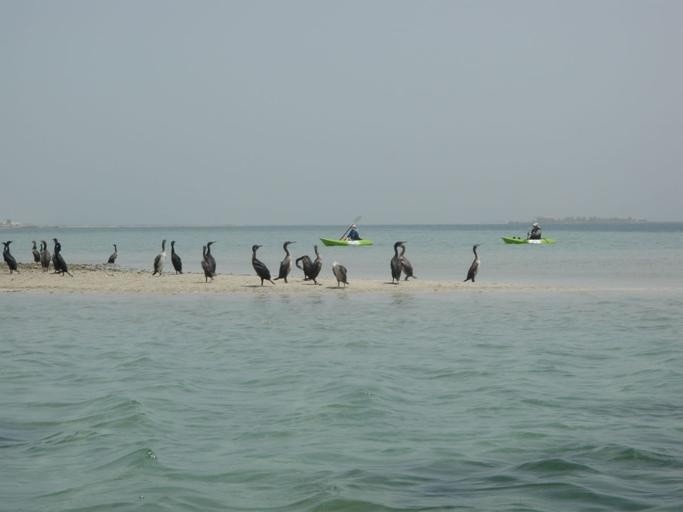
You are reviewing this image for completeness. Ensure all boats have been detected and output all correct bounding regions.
[501,235,557,245]
[319,237,373,247]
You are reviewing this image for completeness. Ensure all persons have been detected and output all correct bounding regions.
[527,222,541,240]
[344,224,361,240]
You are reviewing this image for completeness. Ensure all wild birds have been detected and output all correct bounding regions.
[250,240,322,287]
[6,240,18,271]
[391,241,407,284]
[206,241,217,274]
[1,242,15,274]
[462,243,482,282]
[400,245,418,281]
[201,245,217,282]
[171,240,184,275]
[105,244,118,266]
[31,238,75,277]
[152,239,167,277]
[333,260,350,288]
